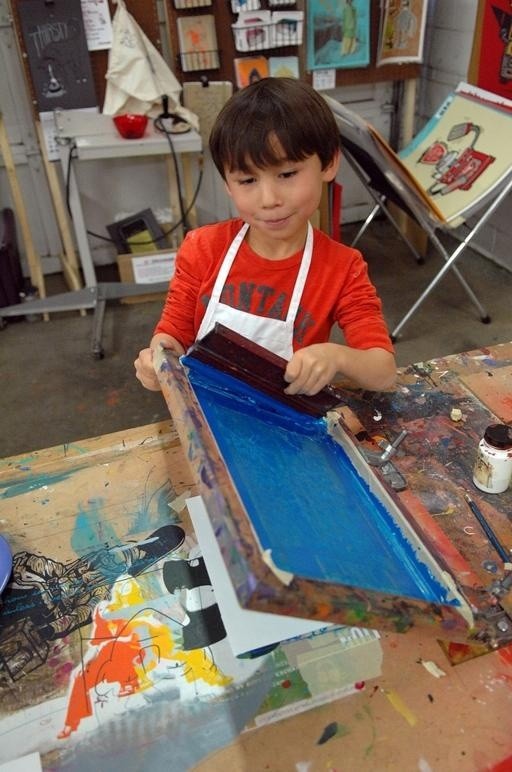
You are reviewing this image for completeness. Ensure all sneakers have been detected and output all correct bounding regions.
[126,525,186,578]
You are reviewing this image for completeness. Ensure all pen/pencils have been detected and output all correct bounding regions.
[463,493,508,563]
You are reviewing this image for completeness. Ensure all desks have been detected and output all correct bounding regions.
[3,336,512,772]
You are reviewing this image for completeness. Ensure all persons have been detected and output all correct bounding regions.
[134,76,397,397]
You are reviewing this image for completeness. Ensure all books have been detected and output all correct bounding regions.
[177,14,221,73]
[173,0,212,9]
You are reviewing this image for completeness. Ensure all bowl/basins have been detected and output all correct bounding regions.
[113,112,150,139]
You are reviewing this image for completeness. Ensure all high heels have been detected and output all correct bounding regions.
[118,679,140,697]
[56,723,77,739]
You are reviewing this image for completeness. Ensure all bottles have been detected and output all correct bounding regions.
[474,422,512,495]
[19,276,41,323]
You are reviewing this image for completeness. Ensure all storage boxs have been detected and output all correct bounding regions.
[117,223,184,304]
